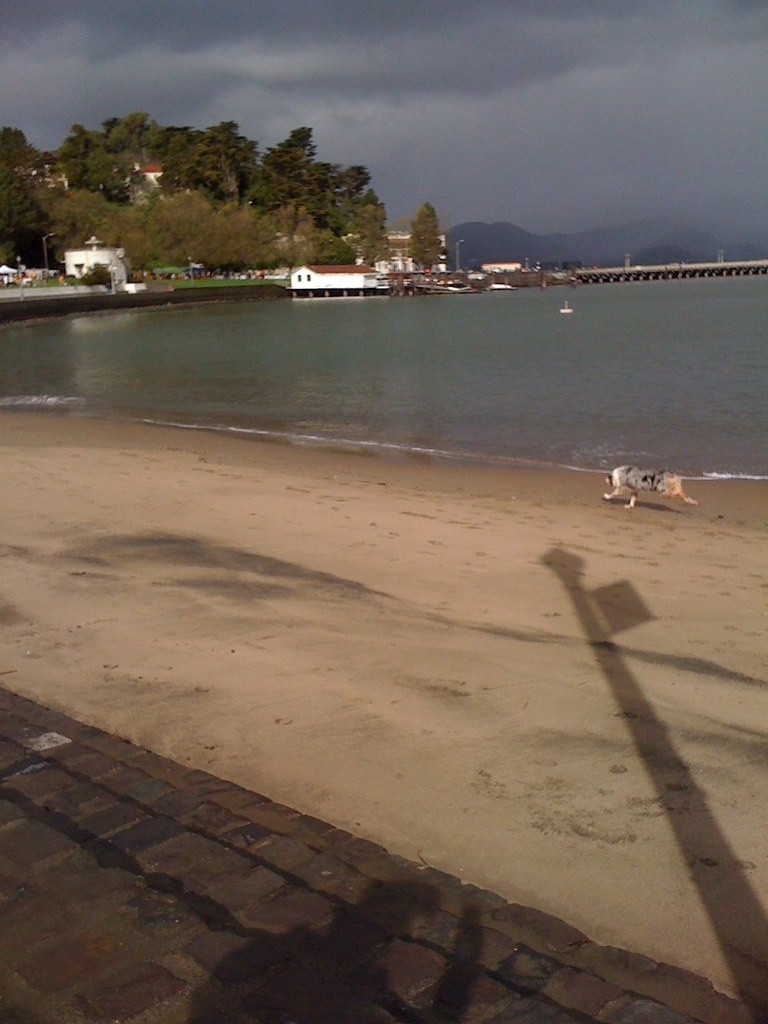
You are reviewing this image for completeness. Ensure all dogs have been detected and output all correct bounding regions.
[601,465,699,510]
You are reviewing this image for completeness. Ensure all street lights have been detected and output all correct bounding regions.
[455,239,465,270]
[41,232,55,286]
